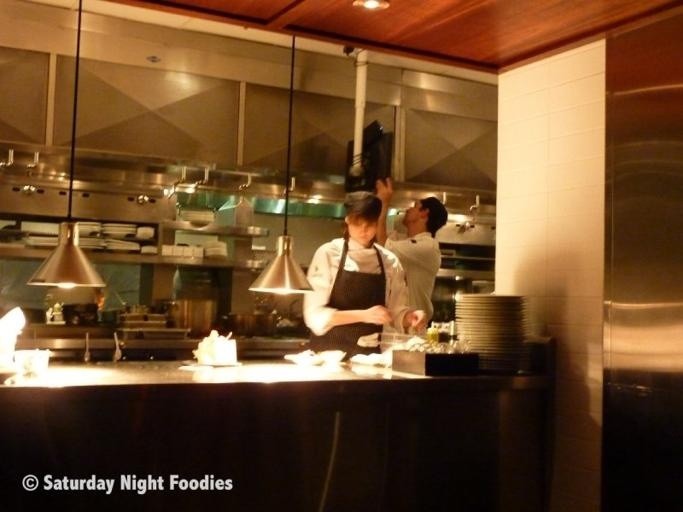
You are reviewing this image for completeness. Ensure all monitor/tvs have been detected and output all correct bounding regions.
[345,132,393,192]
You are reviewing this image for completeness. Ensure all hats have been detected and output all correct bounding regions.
[344,191,381,213]
[424,197,447,230]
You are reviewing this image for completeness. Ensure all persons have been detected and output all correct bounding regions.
[301,191,428,360]
[375,178,447,329]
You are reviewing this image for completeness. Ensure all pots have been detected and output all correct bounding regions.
[164,299,277,339]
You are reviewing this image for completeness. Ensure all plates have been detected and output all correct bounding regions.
[203,241,227,259]
[454,293,527,373]
[20,222,140,252]
[179,212,214,227]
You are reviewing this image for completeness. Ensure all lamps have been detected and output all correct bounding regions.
[25,0,104,292]
[245,31,318,297]
